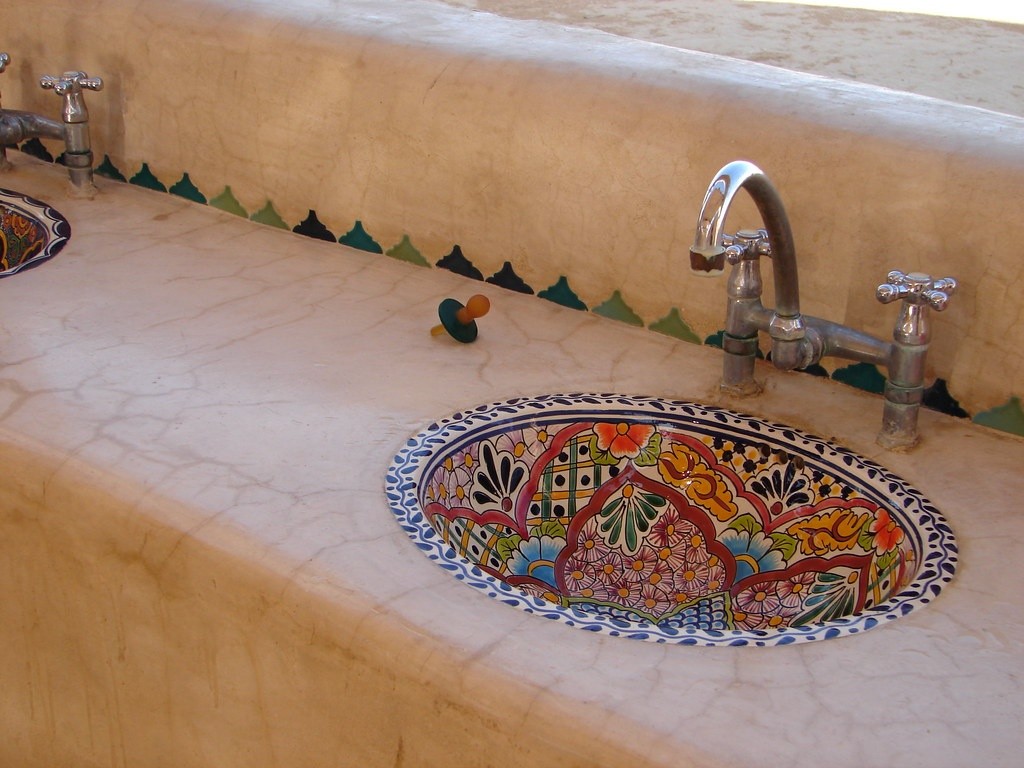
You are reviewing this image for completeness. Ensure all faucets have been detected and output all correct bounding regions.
[688,159,808,373]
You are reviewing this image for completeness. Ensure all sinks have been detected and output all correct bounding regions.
[0,187,72,278]
[384,391,960,647]
